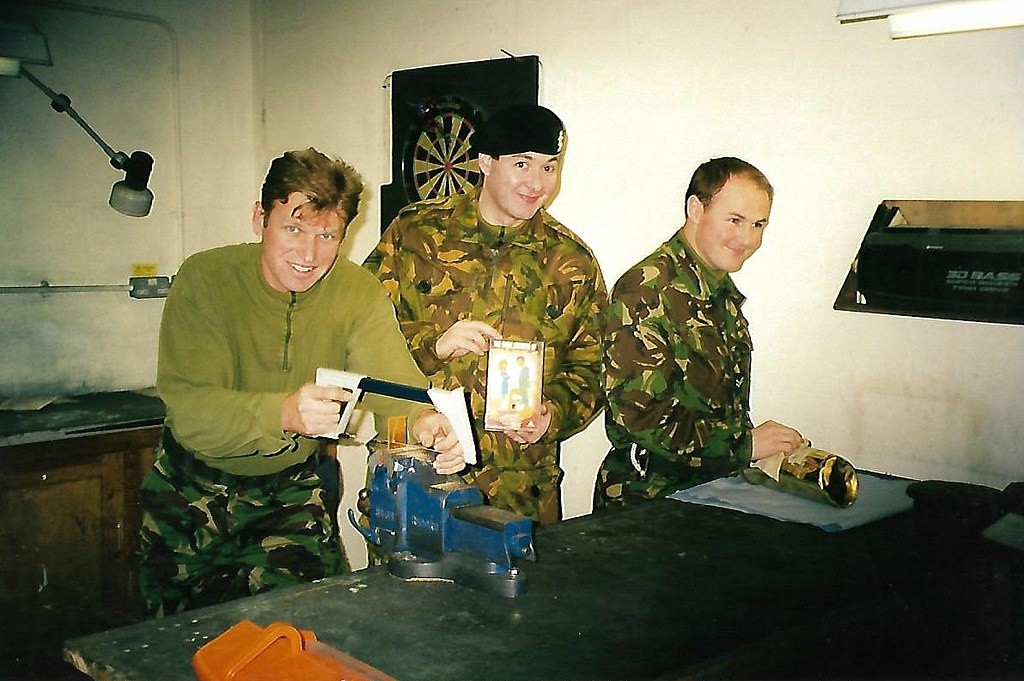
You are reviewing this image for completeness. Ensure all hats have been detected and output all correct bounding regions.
[468,104,565,157]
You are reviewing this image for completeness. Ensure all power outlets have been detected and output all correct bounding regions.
[129,277,169,299]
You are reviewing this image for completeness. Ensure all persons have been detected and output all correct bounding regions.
[128,145,472,626]
[358,103,610,571]
[591,153,804,515]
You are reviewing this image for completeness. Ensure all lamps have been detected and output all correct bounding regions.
[0,58,154,217]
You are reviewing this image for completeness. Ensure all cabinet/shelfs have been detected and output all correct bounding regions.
[1,427,163,652]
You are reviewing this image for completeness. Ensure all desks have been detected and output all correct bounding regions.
[59,466,1024,681]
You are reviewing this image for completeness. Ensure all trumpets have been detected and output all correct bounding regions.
[746,443,859,508]
